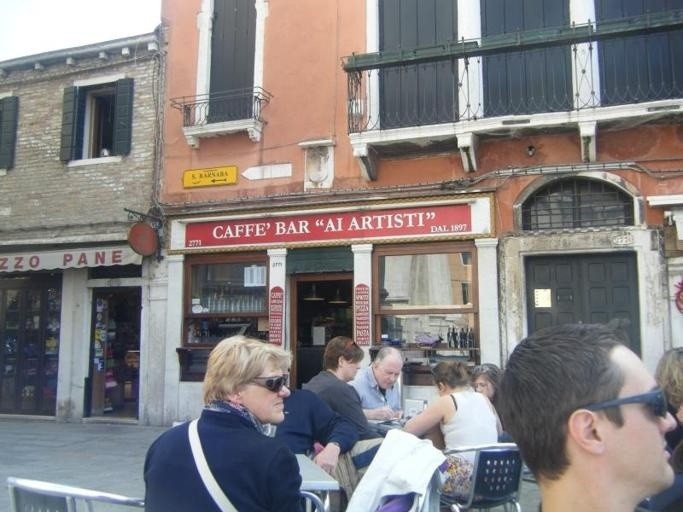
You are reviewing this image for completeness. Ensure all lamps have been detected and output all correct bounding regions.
[328,287,347,303]
[303,283,324,300]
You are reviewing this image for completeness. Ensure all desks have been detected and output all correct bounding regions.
[295,453,339,512]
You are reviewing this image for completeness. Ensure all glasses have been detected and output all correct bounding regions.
[248,373,290,393]
[561,385,669,421]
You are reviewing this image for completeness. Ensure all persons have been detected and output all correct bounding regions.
[297,336,383,441]
[490,319,679,510]
[635,344,683,511]
[468,362,513,443]
[400,358,502,510]
[342,343,406,425]
[274,382,384,511]
[446,325,478,348]
[138,333,305,510]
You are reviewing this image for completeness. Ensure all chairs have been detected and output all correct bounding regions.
[451,443,523,512]
